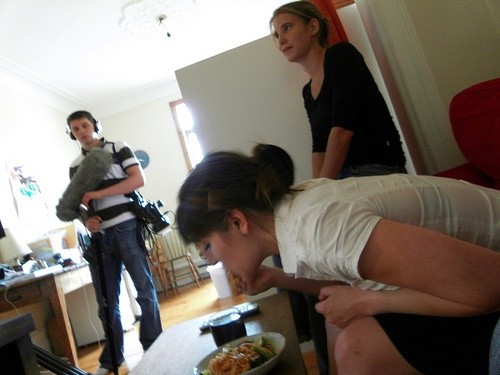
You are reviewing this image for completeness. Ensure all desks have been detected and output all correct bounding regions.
[0,246,149,375]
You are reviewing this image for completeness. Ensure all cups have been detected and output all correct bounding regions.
[207,306,247,348]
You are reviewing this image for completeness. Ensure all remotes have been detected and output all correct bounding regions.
[200,301,260,330]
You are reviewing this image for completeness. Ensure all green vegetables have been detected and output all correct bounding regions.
[251,335,276,361]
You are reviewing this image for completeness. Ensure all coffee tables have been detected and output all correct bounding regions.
[126,291,307,374]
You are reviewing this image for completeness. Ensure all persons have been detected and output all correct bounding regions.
[176,144,500,375]
[66,109,162,375]
[270,1,408,178]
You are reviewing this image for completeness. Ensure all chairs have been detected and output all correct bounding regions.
[152,230,204,298]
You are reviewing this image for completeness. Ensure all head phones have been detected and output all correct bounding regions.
[70,119,99,141]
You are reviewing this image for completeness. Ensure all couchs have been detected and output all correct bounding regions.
[431,77,500,190]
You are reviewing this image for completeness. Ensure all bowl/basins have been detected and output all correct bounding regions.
[193,331,285,375]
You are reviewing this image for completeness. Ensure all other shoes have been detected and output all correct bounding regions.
[103,362,130,375]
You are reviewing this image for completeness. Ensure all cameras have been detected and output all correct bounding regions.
[145,203,171,233]
